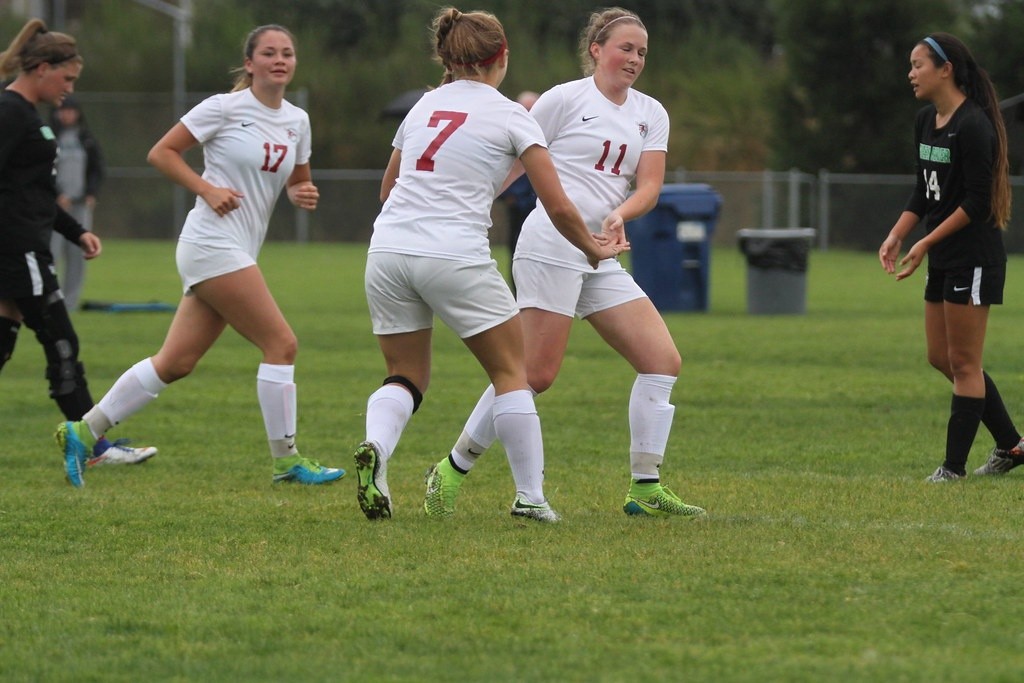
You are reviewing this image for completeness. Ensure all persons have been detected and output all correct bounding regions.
[0,17,158,469]
[54,23,347,486]
[877,32,1024,483]
[420,6,711,522]
[354,7,561,528]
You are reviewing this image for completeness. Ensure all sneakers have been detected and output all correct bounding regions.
[53,419,97,489]
[84,437,158,469]
[353,439,392,520]
[973,438,1024,475]
[623,483,707,519]
[272,457,346,485]
[511,495,563,524]
[925,466,968,483]
[423,463,456,521]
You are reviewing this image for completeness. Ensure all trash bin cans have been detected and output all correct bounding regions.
[627,183,722,310]
[734,226,814,317]
